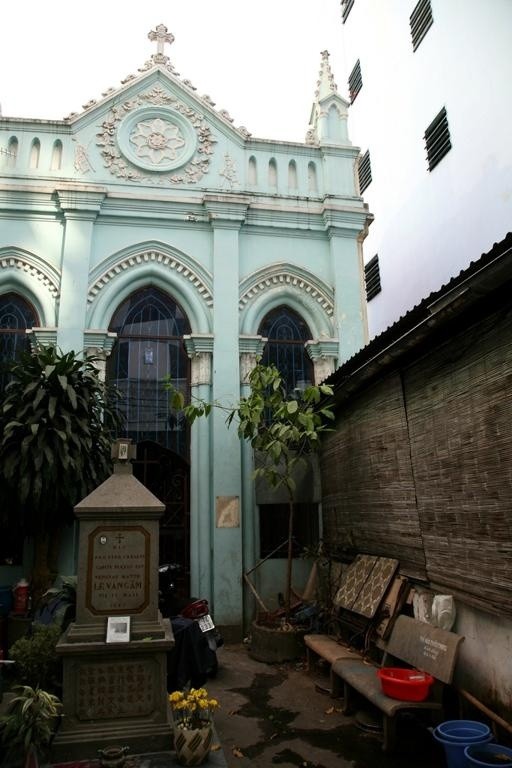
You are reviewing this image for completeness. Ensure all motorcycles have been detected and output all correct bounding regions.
[158,555,225,688]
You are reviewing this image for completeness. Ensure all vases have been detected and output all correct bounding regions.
[172,718,213,768]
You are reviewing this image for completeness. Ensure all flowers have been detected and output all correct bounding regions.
[168,688,223,728]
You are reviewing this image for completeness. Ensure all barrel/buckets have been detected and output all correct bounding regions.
[433,720,512,768]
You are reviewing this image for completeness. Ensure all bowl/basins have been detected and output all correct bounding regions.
[378,666,433,701]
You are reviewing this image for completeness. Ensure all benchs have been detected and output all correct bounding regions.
[331,614,465,750]
[304,617,387,695]
